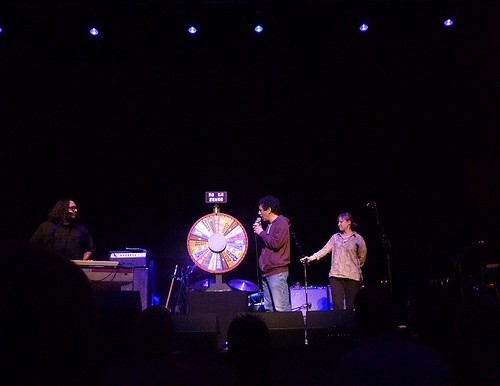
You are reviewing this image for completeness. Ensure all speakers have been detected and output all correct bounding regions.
[173,312,218,349]
[306,309,357,346]
[247,311,305,350]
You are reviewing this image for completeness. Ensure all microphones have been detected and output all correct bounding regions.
[367,201,375,207]
[255,217,261,224]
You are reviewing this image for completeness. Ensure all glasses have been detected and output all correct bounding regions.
[67,206,79,211]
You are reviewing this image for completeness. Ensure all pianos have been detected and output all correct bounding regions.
[73,259,136,291]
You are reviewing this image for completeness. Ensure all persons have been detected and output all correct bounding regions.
[299,213,367,310]
[0,244,500,385]
[252,196,292,313]
[29,198,90,260]
